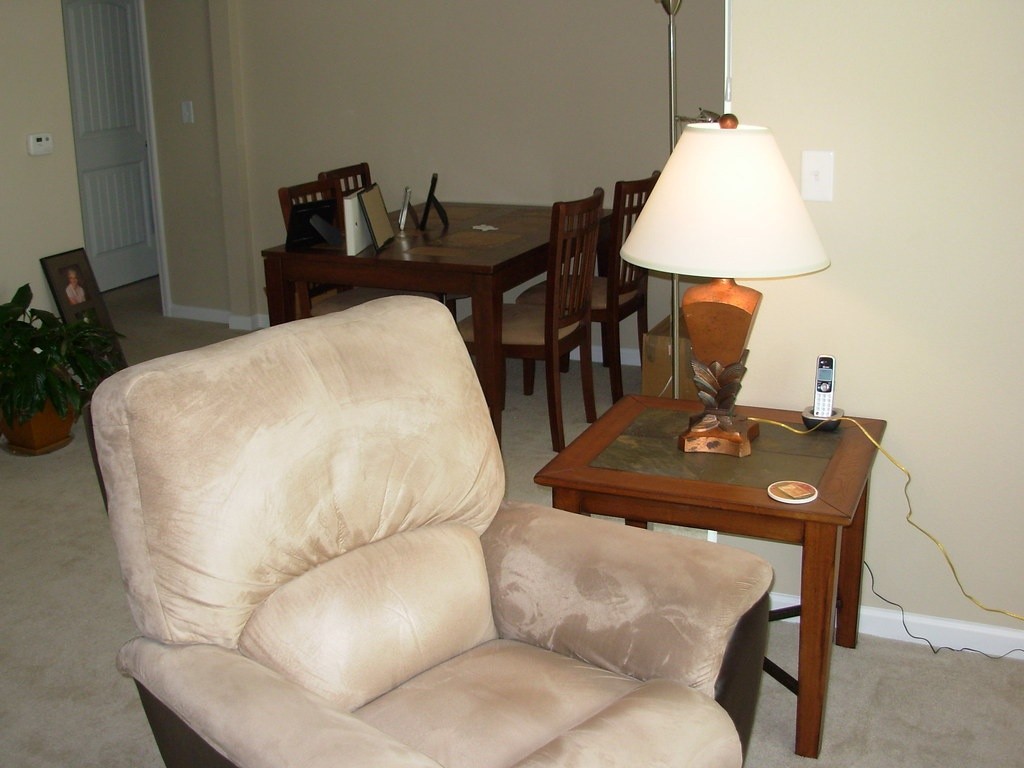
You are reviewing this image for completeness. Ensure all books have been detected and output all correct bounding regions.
[343,186,372,255]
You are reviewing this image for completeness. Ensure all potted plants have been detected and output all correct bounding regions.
[0,285,129,458]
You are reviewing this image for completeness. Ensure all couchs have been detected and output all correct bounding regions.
[82,295,776,768]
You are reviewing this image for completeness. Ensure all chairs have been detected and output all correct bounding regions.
[513,168,661,405]
[277,161,375,244]
[455,187,606,453]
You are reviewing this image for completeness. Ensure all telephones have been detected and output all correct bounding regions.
[801,354,844,432]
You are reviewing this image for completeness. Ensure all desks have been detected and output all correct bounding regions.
[261,197,614,454]
[531,393,887,761]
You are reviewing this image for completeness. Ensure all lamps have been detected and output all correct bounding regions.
[617,113,831,459]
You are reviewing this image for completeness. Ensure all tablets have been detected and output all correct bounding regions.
[358,182,395,252]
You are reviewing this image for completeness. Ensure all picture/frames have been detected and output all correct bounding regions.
[38,248,116,333]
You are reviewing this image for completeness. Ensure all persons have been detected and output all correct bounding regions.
[65,269,85,304]
